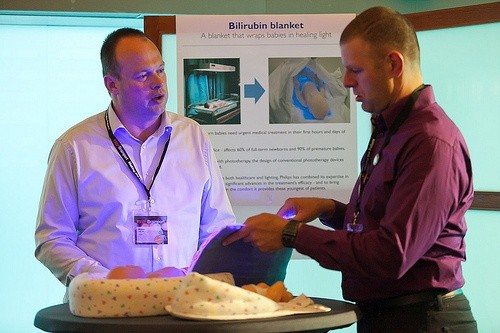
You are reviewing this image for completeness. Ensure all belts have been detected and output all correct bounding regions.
[356,287,463,311]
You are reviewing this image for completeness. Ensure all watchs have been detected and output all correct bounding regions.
[278,219,301,249]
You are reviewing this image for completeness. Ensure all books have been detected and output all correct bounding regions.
[176,223,295,287]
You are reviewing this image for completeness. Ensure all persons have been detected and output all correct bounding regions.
[33,28,236,300]
[291,68,331,120]
[222,5,479,332]
[107,263,189,282]
[239,281,294,302]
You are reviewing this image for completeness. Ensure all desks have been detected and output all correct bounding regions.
[32,295,358,333]
